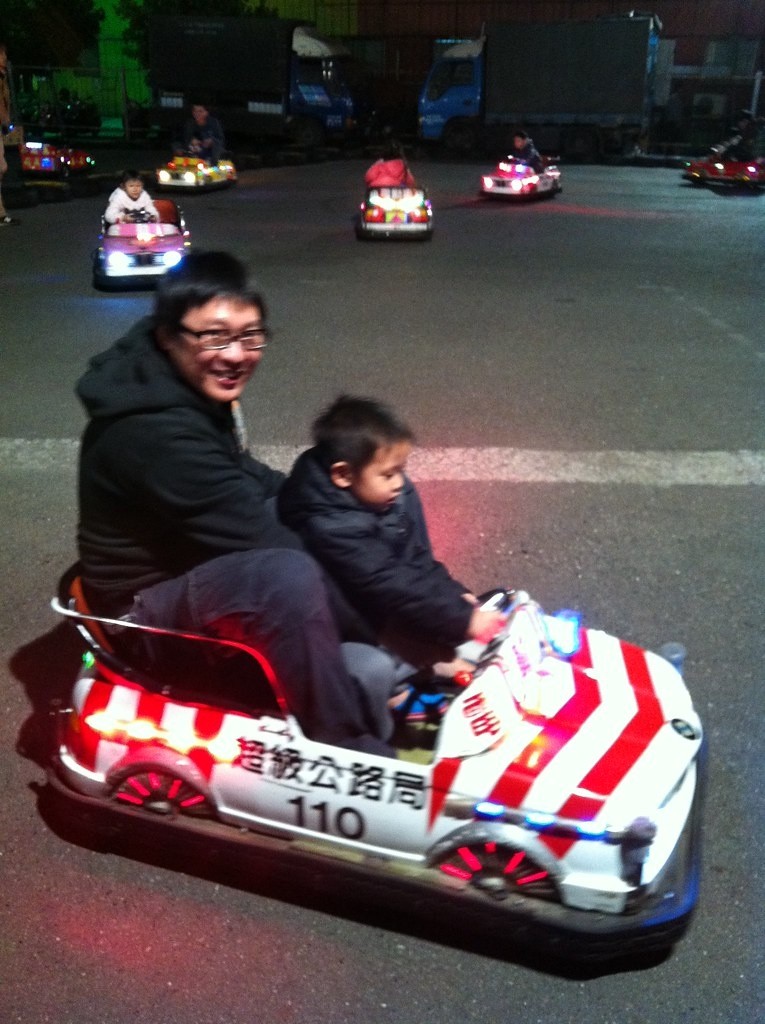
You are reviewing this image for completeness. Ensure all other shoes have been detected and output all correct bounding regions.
[386,685,447,719]
[338,733,397,758]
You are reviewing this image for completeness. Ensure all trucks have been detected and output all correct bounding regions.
[138,13,379,144]
[417,9,662,157]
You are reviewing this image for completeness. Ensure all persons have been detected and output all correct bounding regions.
[505,131,543,173]
[74,252,400,759]
[175,101,222,165]
[276,389,507,730]
[716,105,765,162]
[103,166,160,224]
[365,143,417,190]
[0,46,22,228]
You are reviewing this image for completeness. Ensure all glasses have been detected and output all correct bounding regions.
[179,324,272,350]
